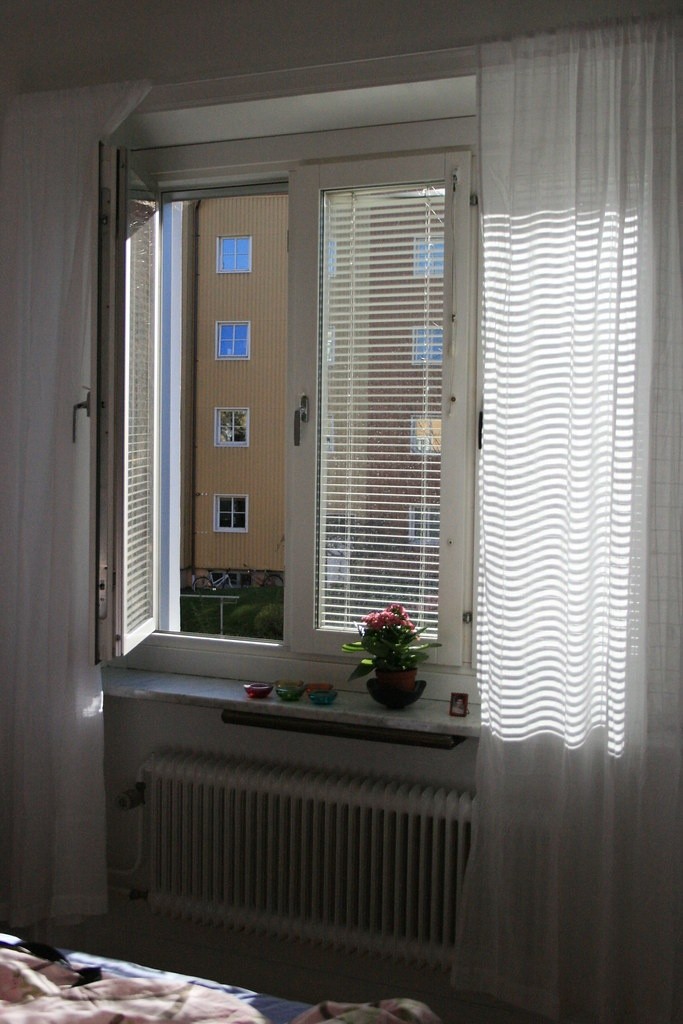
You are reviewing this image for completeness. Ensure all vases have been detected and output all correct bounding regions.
[366,669,426,710]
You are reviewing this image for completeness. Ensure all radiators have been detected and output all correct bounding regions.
[116,746,555,993]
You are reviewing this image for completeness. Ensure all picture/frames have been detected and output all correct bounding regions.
[449,690,468,719]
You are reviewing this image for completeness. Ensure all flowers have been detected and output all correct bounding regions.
[342,602,442,682]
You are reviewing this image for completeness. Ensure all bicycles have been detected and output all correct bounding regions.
[241,563,284,587]
[191,566,242,593]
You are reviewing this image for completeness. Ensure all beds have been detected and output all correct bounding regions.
[0,934,446,1024]
